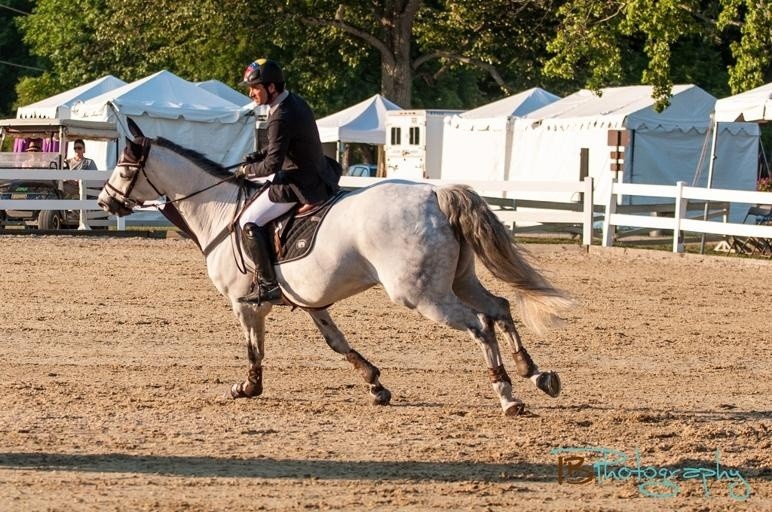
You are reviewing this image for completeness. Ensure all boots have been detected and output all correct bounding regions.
[237,222,284,305]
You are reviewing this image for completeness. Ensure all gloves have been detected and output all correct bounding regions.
[234,150,262,179]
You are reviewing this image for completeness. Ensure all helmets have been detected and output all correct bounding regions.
[238,58,283,86]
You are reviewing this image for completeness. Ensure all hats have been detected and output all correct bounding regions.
[24,141,44,151]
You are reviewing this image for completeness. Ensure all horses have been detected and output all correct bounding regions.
[97,116,572,416]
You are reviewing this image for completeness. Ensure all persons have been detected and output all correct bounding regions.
[232,58,343,305]
[24,140,47,220]
[61,138,112,229]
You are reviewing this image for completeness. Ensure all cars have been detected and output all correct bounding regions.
[347,162,379,177]
